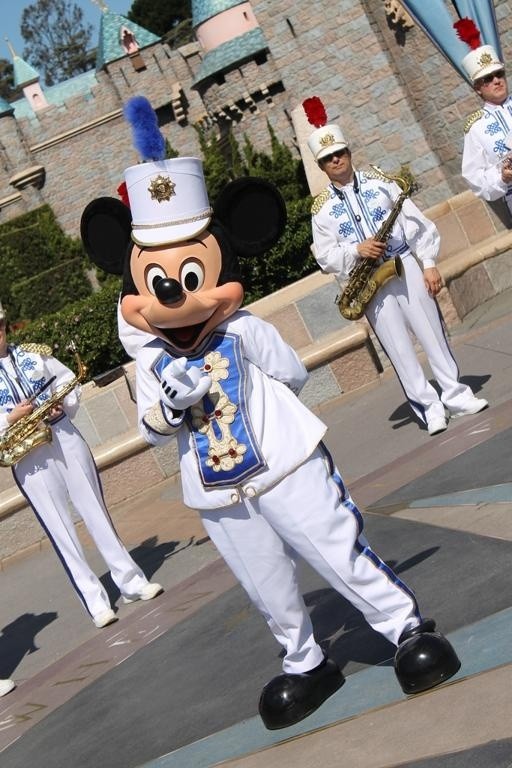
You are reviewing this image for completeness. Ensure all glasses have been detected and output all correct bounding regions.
[476,68,505,89]
[321,148,348,164]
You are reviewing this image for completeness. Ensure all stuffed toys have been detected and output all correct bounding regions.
[77,97,463,733]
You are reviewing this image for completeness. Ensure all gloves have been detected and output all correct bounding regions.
[157,354,214,413]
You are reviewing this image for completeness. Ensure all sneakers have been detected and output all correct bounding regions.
[425,403,450,437]
[447,396,489,418]
[0,677,16,698]
[122,581,165,605]
[92,608,119,629]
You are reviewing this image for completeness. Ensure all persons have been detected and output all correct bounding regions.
[315,145,490,438]
[462,66,511,224]
[0,302,164,631]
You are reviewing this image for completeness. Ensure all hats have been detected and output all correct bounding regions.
[122,154,218,249]
[303,123,352,164]
[459,43,506,86]
[0,303,6,321]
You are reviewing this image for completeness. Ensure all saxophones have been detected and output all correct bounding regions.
[335,162,420,320]
[0,338,85,467]
